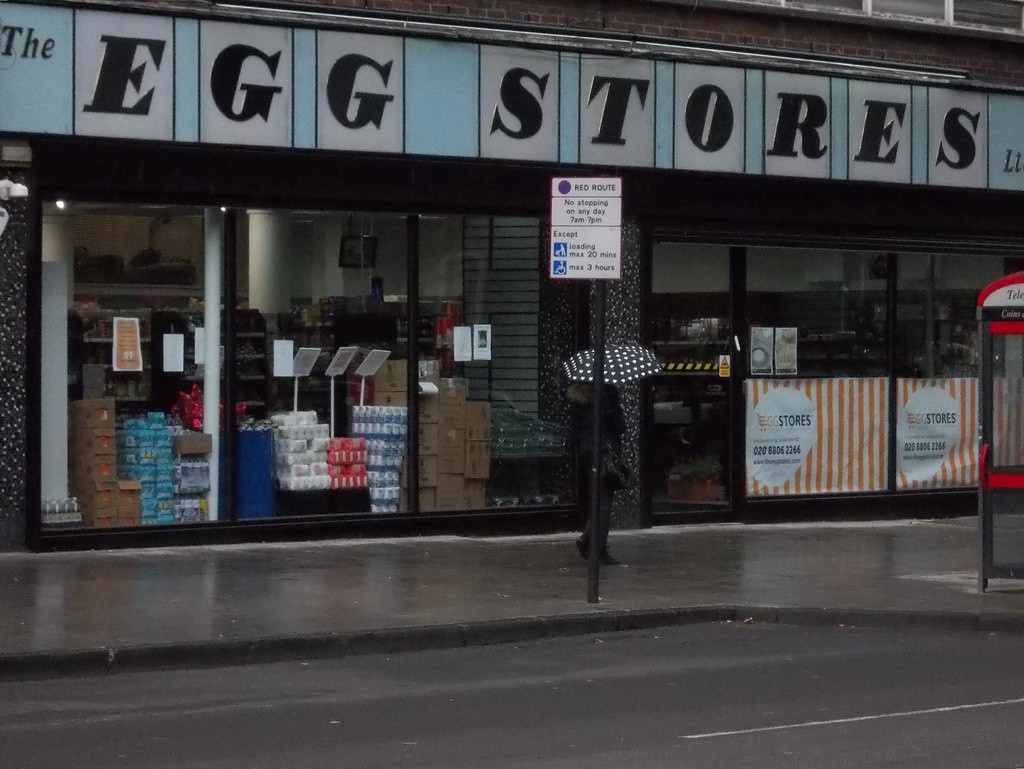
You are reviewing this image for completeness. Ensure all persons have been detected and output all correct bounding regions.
[569,380,629,566]
[67,308,87,402]
[832,320,923,378]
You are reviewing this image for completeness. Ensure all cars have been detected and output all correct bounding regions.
[654,390,724,427]
[907,342,976,376]
[309,379,370,420]
[832,342,886,375]
[469,383,570,460]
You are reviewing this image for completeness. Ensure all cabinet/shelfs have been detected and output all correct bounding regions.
[652,338,884,442]
[72,283,444,407]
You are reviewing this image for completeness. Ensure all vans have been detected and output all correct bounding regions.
[778,343,828,361]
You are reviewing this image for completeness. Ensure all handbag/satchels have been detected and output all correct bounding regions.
[606,450,628,492]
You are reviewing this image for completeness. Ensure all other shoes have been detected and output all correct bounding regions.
[576,536,590,559]
[599,546,620,565]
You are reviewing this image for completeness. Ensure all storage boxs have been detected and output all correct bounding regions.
[374,359,440,391]
[399,456,437,488]
[439,382,467,406]
[467,401,491,441]
[438,407,466,429]
[397,487,435,512]
[117,481,142,507]
[117,507,140,527]
[69,428,116,455]
[80,509,117,528]
[439,431,465,475]
[172,431,212,455]
[404,424,439,457]
[68,399,116,430]
[435,475,464,511]
[68,483,117,508]
[465,441,490,479]
[373,390,439,423]
[464,478,486,510]
[68,456,116,483]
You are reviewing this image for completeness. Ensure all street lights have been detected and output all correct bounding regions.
[840,284,850,329]
[871,255,893,373]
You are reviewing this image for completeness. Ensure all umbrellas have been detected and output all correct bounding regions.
[562,342,663,386]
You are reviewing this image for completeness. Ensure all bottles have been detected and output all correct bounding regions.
[42,497,79,526]
